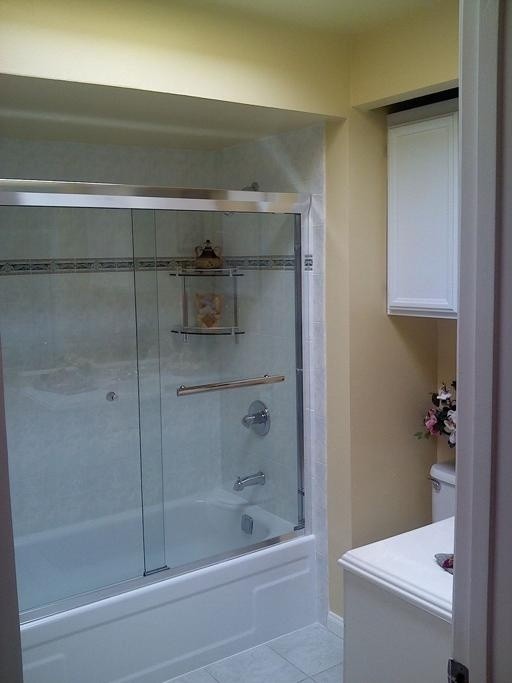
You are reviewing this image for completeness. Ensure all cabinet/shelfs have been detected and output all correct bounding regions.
[380,95,461,321]
[167,263,248,342]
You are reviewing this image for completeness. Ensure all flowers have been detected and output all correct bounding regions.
[410,370,457,450]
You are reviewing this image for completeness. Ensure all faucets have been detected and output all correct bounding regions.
[232,472,267,493]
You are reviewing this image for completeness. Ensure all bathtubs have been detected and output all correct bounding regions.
[9,489,318,682]
